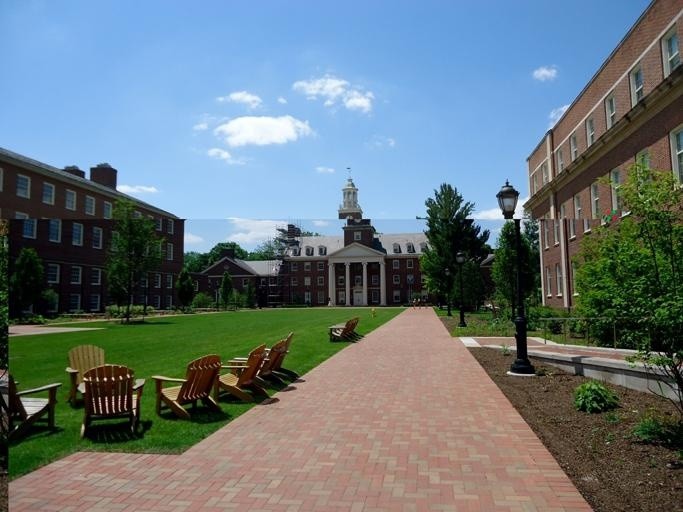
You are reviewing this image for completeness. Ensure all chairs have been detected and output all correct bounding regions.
[327,316,360,342]
[8,331,294,438]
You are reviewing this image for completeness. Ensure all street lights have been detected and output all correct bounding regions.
[442,266,453,317]
[494,175,541,379]
[453,248,473,330]
[437,281,442,312]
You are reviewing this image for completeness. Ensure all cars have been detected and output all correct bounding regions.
[484,303,500,310]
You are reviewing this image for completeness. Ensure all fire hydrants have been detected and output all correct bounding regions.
[369,308,377,319]
